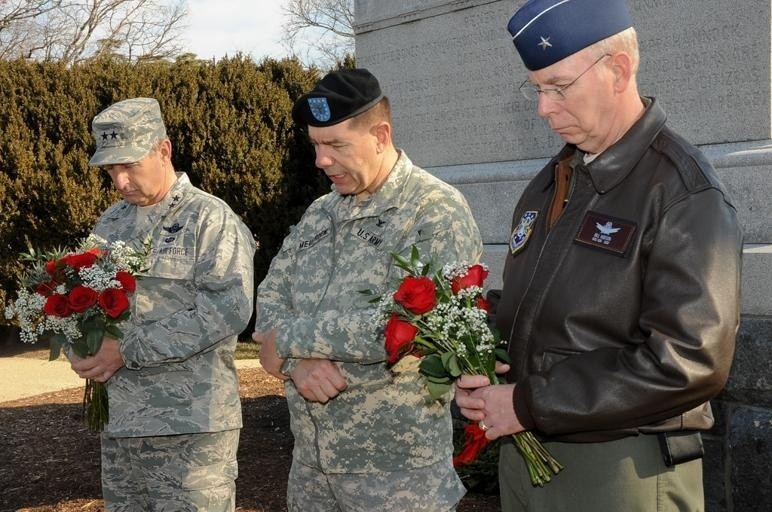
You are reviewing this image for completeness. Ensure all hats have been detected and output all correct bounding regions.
[88,97,167,166]
[507,0,634,72]
[292,68,383,127]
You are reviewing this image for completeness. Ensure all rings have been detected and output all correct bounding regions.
[478,421,488,431]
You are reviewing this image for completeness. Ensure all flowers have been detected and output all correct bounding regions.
[358,244,563,487]
[3,237,157,436]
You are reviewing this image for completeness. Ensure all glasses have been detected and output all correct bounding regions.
[519,54,612,101]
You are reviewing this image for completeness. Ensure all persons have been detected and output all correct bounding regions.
[248,67,488,510]
[452,0,744,512]
[62,94,257,512]
